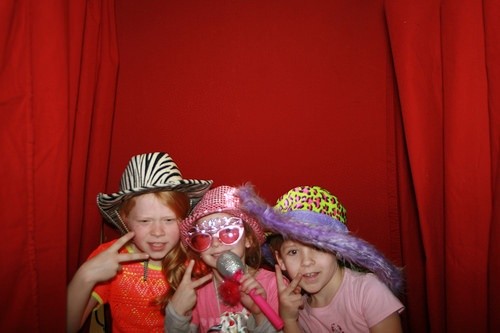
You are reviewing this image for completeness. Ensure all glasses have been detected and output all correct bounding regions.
[185,216,244,251]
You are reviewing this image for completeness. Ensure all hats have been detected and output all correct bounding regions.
[234,182,405,297]
[179,185,265,257]
[97,152,213,235]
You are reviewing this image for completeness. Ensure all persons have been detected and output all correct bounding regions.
[236,185,405,333]
[64,151,214,333]
[150,185,302,333]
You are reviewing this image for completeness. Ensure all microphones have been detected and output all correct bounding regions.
[215,251,285,331]
